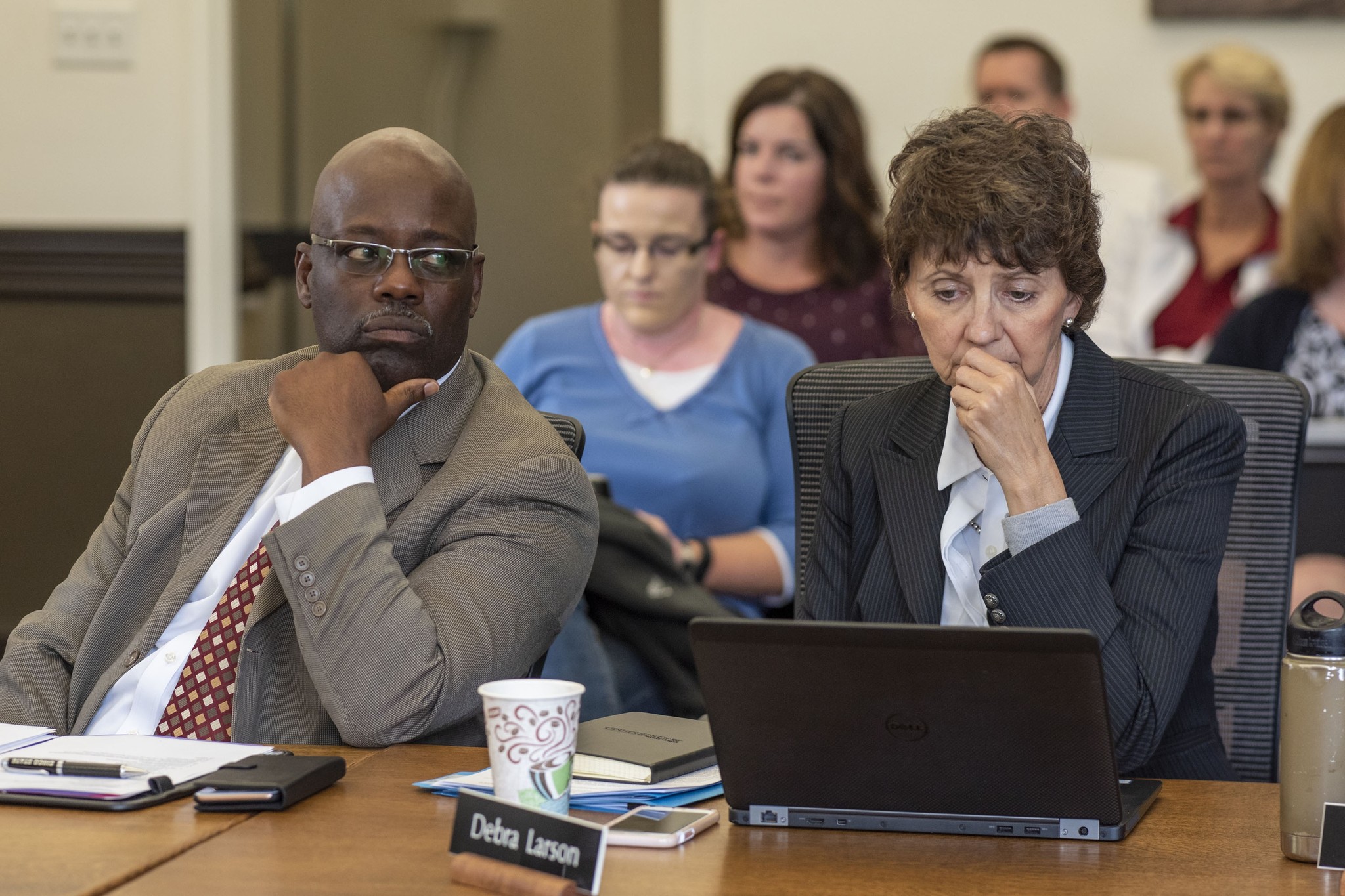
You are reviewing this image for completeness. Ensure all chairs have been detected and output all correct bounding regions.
[524,406,587,681]
[783,354,1315,786]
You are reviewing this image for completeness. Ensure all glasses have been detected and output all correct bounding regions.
[310,233,479,282]
[592,232,710,257]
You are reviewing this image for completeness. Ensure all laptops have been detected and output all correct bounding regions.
[688,618,1162,840]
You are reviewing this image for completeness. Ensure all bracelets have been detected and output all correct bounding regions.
[691,535,710,583]
[681,541,694,581]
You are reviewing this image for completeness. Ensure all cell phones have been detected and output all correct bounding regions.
[603,804,720,848]
[193,754,346,812]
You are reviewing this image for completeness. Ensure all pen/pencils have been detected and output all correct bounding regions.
[0,758,147,778]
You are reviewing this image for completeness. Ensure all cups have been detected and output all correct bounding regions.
[477,677,586,817]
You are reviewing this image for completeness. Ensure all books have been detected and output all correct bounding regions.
[570,711,717,785]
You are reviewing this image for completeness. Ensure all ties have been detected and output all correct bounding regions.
[154,519,280,743]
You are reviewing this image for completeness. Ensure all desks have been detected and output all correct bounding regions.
[0,743,1342,896]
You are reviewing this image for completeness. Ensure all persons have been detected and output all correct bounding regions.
[490,31,1344,723]
[0,125,597,751]
[790,106,1242,782]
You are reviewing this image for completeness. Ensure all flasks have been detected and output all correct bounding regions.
[1275,594,1345,866]
